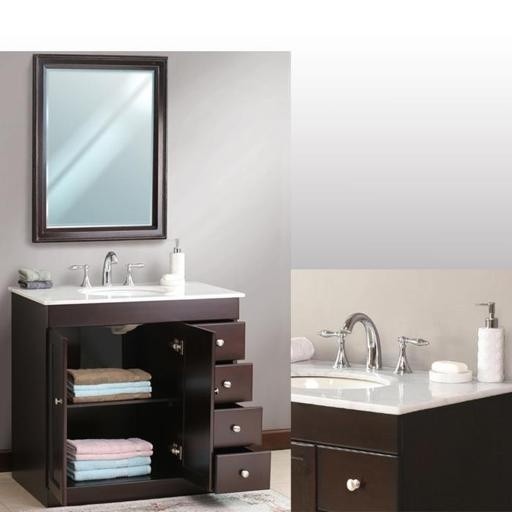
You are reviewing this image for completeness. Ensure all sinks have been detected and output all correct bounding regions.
[290,366,398,390]
[81,287,168,296]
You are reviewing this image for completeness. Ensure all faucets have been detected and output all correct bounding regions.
[339,311,384,369]
[101,251,119,286]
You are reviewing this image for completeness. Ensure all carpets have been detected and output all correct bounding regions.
[37,488,290,512]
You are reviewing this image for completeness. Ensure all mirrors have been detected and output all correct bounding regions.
[31,55,170,242]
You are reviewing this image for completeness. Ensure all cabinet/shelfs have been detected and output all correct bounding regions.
[7,280,273,508]
[291,392,511,512]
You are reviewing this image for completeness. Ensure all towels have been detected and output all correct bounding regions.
[66,366,153,403]
[18,268,52,289]
[65,436,154,482]
